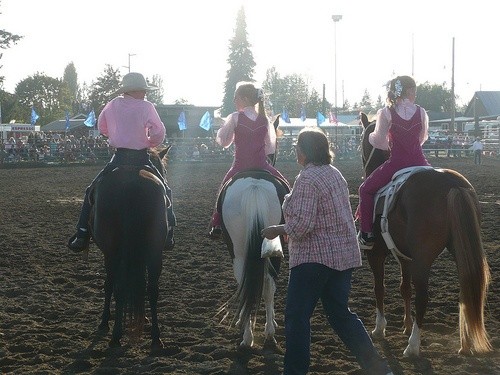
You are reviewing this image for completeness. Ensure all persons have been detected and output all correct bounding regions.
[69,72,178,254]
[353,76,429,250]
[207,84,293,238]
[1,131,485,167]
[251,128,389,375]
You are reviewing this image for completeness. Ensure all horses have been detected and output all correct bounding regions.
[358,110,494,363]
[213,113,293,352]
[86,140,170,354]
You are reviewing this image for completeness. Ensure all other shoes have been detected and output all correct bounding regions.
[71,232,87,250]
[355,229,375,250]
[165,236,175,250]
[210,224,223,238]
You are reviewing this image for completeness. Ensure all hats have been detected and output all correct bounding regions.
[111,72,159,98]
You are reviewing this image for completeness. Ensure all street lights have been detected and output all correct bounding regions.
[331,14,343,145]
[121,53,137,72]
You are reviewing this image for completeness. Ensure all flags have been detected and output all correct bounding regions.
[30,106,338,140]
